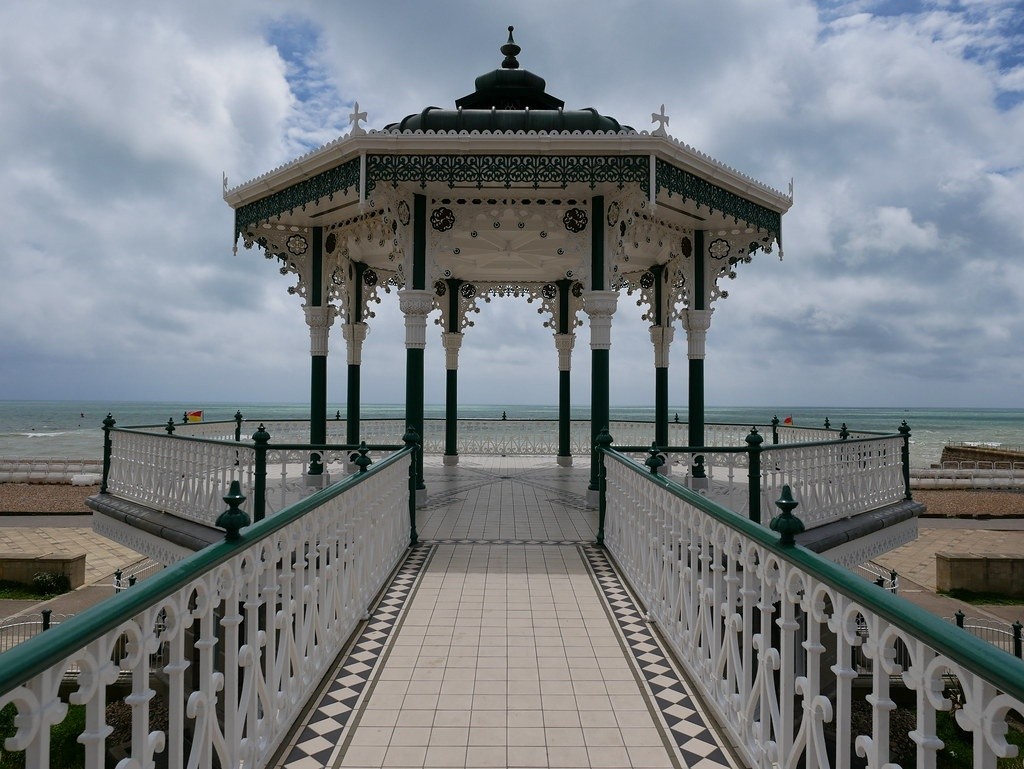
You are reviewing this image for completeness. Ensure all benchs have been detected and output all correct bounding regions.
[0,552,87,592]
[933,551,1024,598]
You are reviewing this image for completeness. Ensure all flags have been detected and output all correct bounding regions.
[185,410,203,422]
[784,415,793,425]
